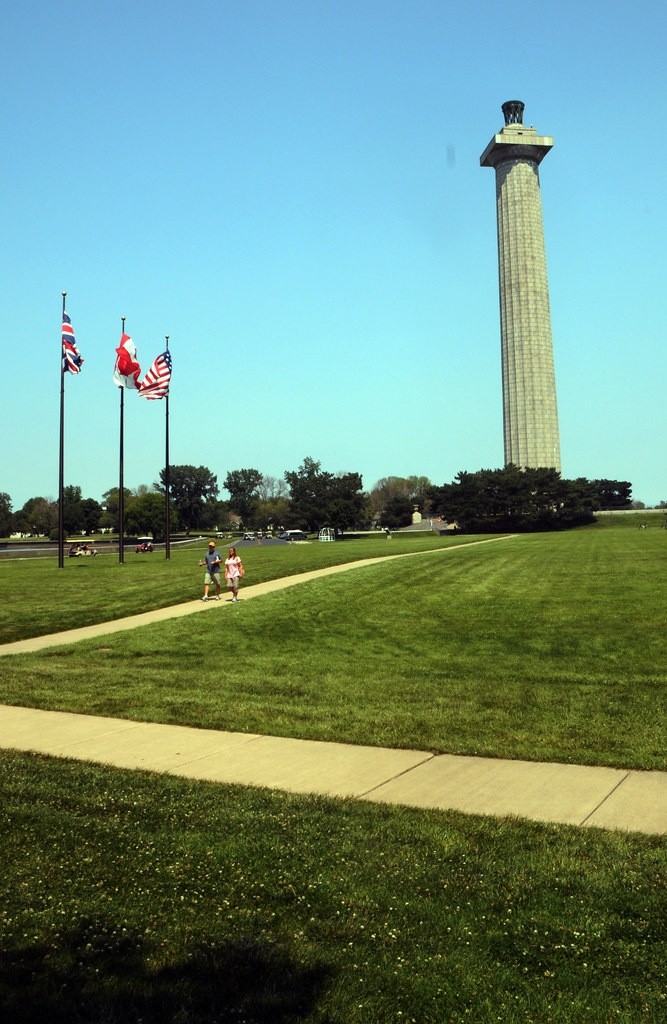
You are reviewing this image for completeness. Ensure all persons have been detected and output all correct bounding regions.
[142,539,151,547]
[199,541,223,602]
[224,547,244,602]
[71,543,91,549]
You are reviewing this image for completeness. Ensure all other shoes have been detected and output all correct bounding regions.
[232,594,237,602]
[201,595,209,602]
[216,594,220,600]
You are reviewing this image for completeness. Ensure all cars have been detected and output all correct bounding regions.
[276,530,302,540]
[284,533,307,541]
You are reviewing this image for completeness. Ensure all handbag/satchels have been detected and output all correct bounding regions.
[236,556,246,579]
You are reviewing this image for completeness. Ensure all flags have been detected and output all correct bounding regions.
[61,310,85,375]
[112,330,142,391]
[137,349,173,401]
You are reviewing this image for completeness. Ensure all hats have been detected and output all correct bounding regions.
[208,541,216,546]
